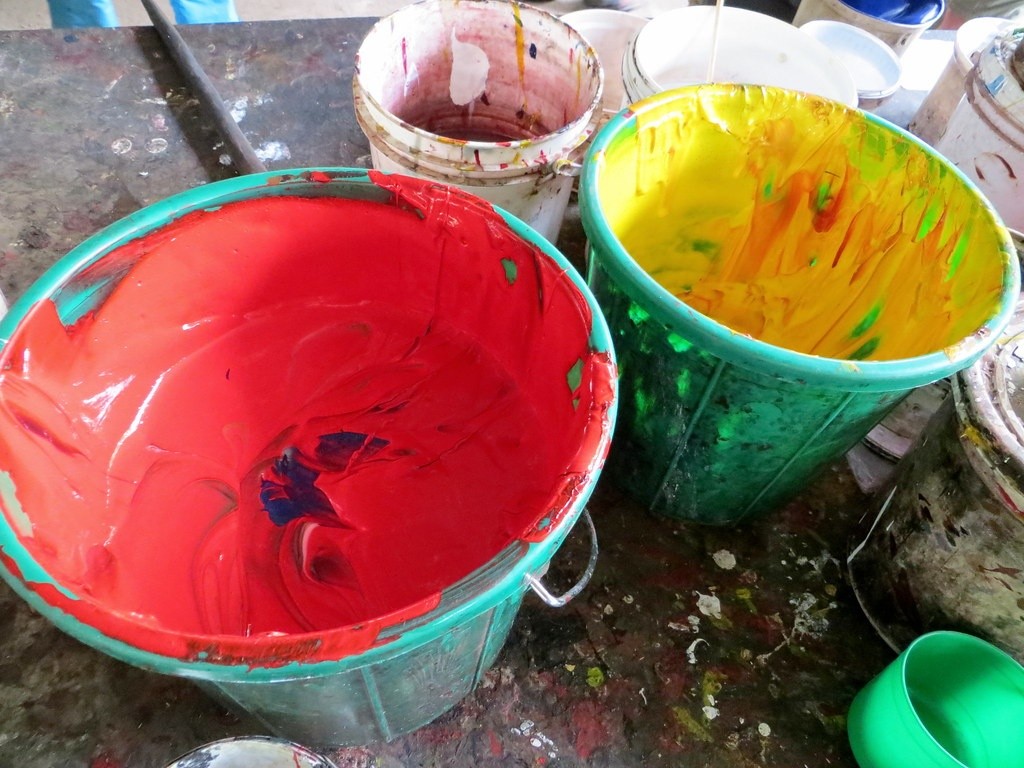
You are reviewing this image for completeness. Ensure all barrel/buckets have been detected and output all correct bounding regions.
[1,167,622,750]
[164,736,338,768]
[621,6,859,110]
[580,84,1022,527]
[908,17,1024,148]
[792,0,946,58]
[558,9,651,191]
[932,24,1024,234]
[353,0,606,247]
[800,20,904,112]
[847,632,1024,768]
[850,323,1024,667]
